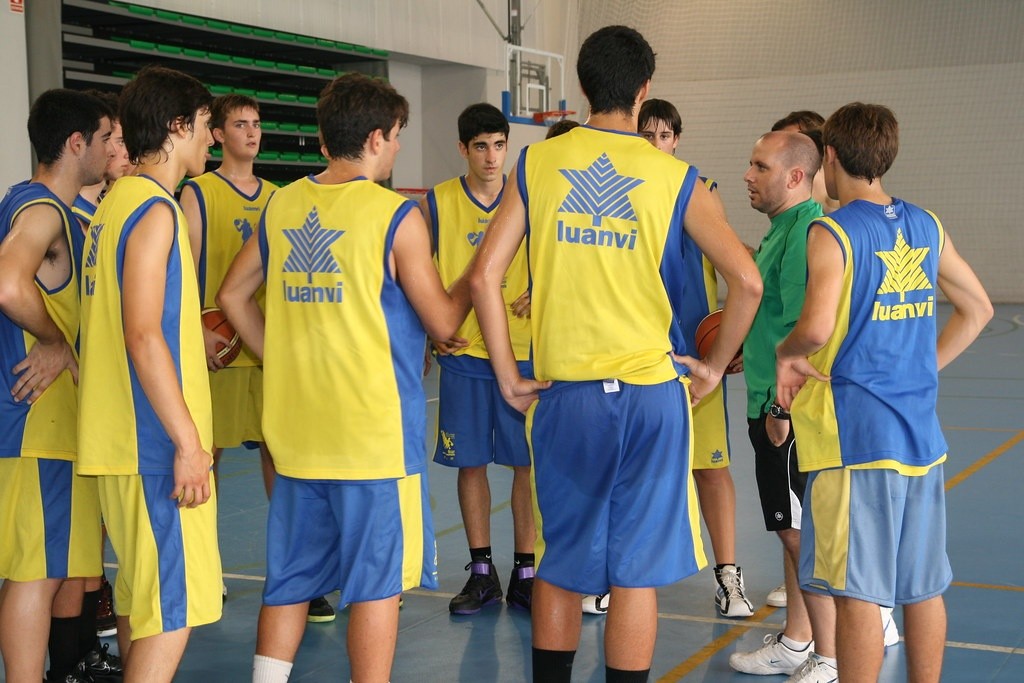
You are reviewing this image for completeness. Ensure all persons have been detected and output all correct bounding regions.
[0,67,405,683]
[418,102,538,614]
[469,25,764,683]
[768,101,996,683]
[213,70,474,683]
[545,97,901,683]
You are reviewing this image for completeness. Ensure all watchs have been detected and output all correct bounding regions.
[769,401,791,420]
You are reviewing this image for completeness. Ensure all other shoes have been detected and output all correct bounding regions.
[307,596,335,622]
[766,584,787,608]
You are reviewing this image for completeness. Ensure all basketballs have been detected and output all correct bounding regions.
[694,308,744,376]
[200,306,243,371]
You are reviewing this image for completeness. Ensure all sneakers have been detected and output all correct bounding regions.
[449,557,503,614]
[713,565,755,616]
[728,632,815,675]
[93,577,117,637]
[582,591,610,614]
[85,636,122,681]
[783,651,839,683]
[46,658,96,683]
[506,561,535,613]
